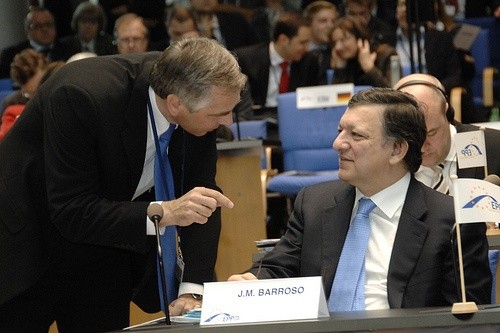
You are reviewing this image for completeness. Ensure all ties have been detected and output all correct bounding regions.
[211,28,217,40]
[279,62,288,94]
[430,162,450,196]
[329,197,377,312]
[154,123,177,311]
[84,46,89,52]
[41,49,49,58]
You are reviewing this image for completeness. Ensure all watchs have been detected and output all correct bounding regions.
[192,294,202,300]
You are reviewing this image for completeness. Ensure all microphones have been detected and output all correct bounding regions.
[147,203,171,326]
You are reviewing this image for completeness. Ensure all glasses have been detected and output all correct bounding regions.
[119,36,146,44]
[33,22,54,29]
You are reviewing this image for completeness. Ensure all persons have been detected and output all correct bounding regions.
[0,0,250,138]
[231,0,500,126]
[0,40,249,333]
[393,73,500,196]
[227,87,492,310]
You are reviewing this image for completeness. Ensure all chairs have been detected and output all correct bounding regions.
[233,118,271,216]
[266,84,374,215]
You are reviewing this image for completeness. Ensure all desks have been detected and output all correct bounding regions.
[217,138,269,280]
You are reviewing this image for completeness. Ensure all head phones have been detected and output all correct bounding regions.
[396,80,455,125]
